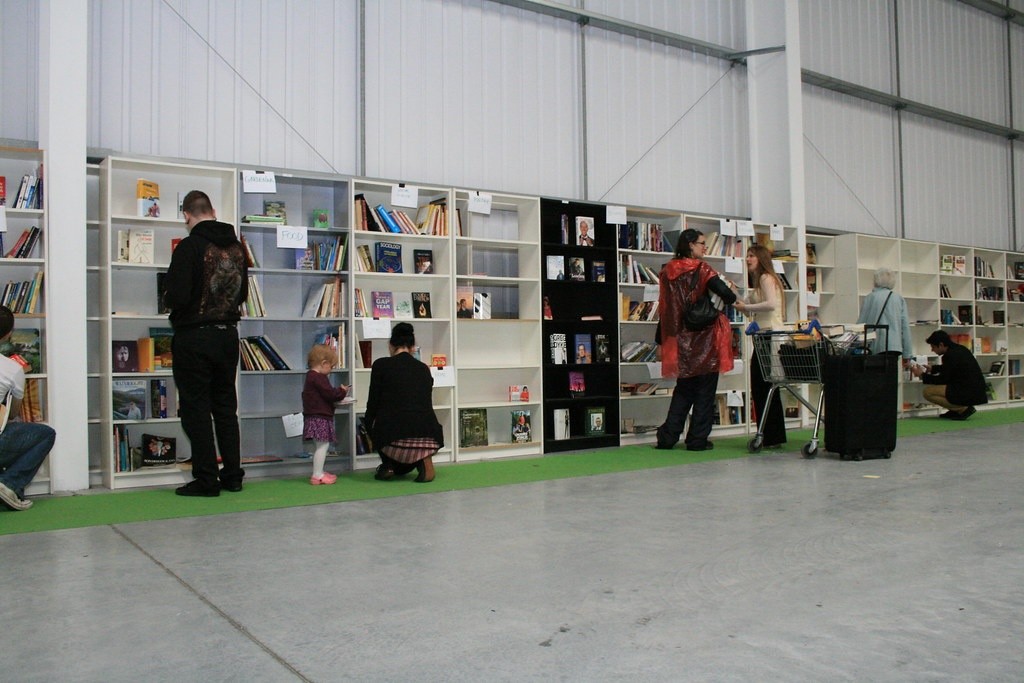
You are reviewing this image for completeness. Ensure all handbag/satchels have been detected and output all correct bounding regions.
[679,261,719,332]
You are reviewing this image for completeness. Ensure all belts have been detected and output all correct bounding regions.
[199,324,237,330]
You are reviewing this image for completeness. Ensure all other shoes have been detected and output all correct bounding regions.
[952,405,977,420]
[374,462,395,480]
[938,410,956,419]
[414,456,436,483]
[686,440,714,451]
[761,443,781,449]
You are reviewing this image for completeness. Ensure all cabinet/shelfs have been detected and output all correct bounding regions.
[0,145,1024,496]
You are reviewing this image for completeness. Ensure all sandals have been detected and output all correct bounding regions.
[309,471,337,485]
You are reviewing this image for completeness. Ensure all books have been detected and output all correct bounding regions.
[240,194,448,451]
[456,209,462,236]
[457,285,491,319]
[0,174,43,375]
[458,384,533,447]
[618,221,746,434]
[113,180,187,473]
[544,215,610,441]
[939,254,1024,400]
[770,243,823,320]
[821,323,875,355]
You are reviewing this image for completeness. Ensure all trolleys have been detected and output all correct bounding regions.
[745,321,874,459]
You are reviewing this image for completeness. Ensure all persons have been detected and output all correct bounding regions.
[565,410,569,437]
[579,221,594,246]
[519,412,525,430]
[0,305,56,510]
[303,321,444,485]
[520,386,529,402]
[577,344,591,364]
[655,227,737,452]
[731,246,787,449]
[127,399,141,420]
[459,299,470,318]
[857,267,988,420]
[162,189,249,497]
[593,417,601,430]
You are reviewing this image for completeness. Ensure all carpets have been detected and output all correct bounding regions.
[0,406,1024,535]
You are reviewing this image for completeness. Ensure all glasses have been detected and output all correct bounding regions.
[693,241,706,246]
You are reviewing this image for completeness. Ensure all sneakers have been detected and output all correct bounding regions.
[175,479,221,497]
[219,477,242,492]
[0,481,34,511]
